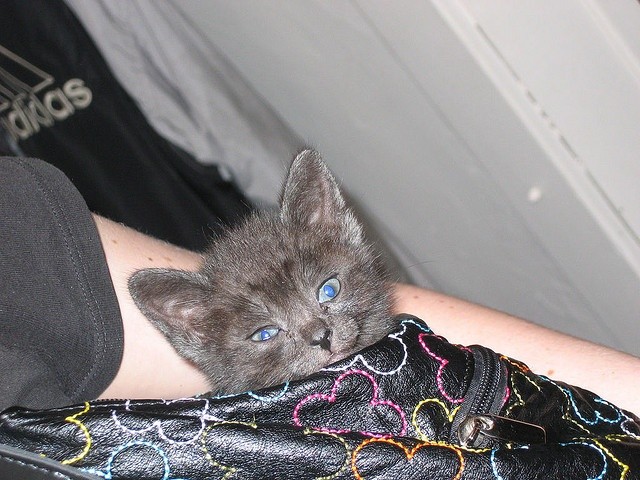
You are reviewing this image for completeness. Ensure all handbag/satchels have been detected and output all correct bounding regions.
[0,314,640,479]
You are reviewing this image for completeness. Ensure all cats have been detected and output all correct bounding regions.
[126,144,393,398]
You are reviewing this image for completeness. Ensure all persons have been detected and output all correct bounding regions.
[0,154,639,419]
